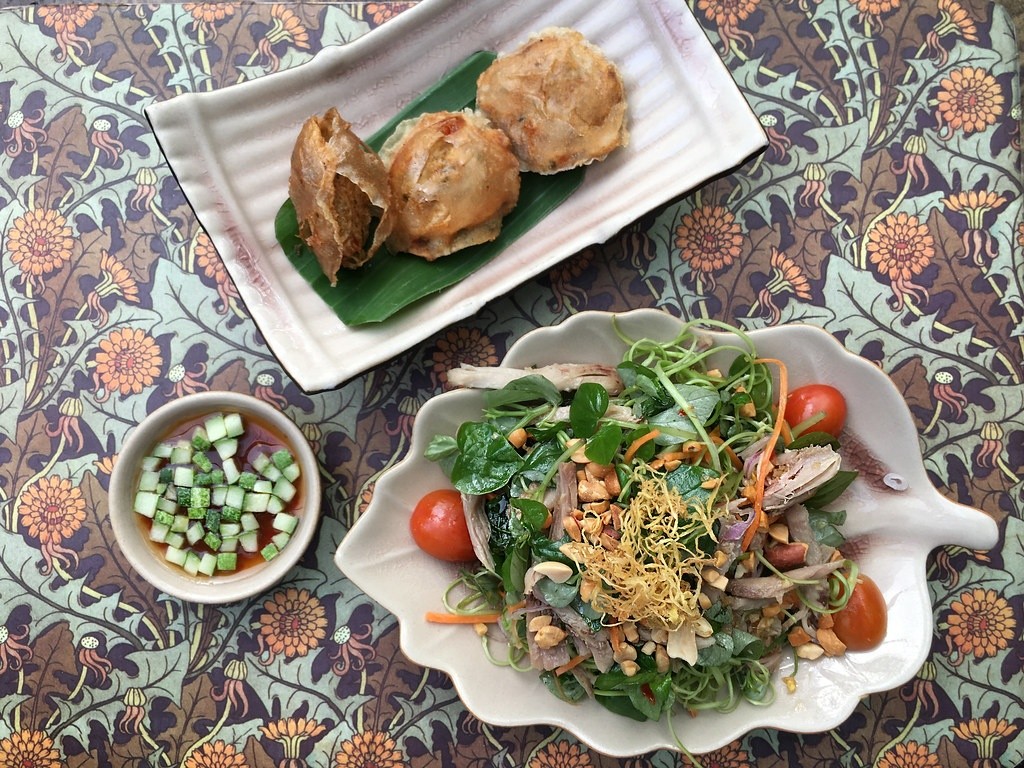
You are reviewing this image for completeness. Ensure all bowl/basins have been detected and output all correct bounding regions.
[108,391,322,605]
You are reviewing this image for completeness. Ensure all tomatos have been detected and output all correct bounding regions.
[830,567,887,647]
[412,489,479,563]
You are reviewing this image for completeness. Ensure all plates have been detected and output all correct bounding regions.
[333,309,1000,758]
[144,1,771,396]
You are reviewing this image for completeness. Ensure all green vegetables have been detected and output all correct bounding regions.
[425,318,866,767]
